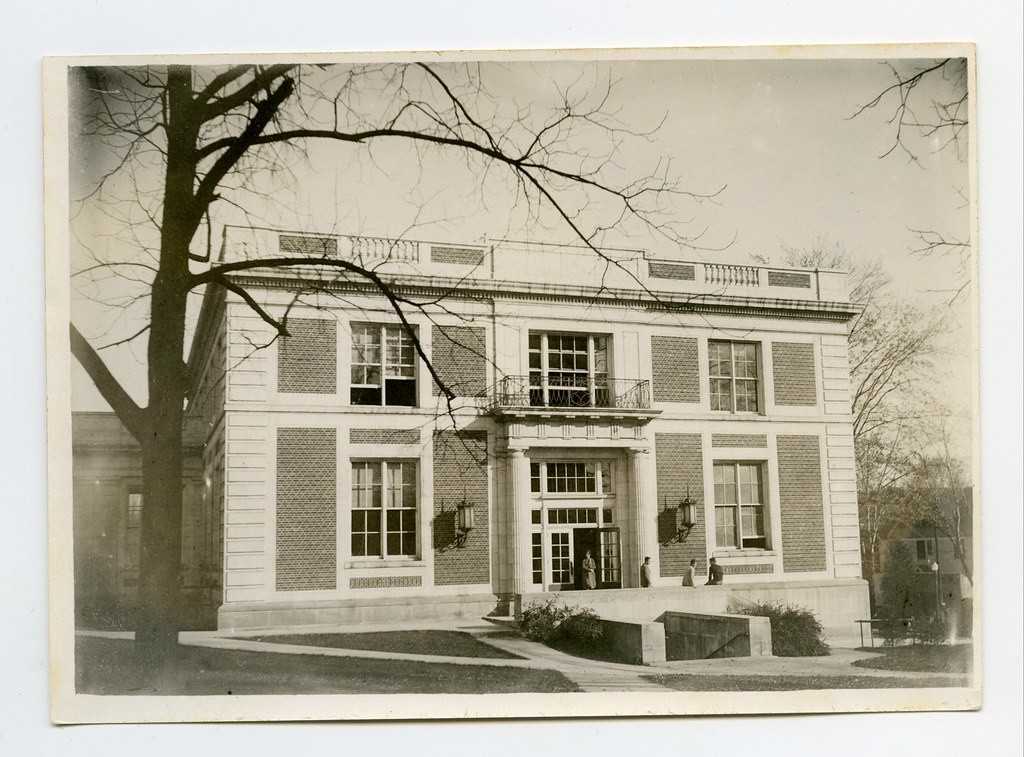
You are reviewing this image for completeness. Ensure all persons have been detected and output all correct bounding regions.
[640,557,652,588]
[681,558,698,589]
[703,557,724,585]
[581,549,596,591]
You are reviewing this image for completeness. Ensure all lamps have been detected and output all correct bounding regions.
[456,487,475,548]
[679,483,698,544]
